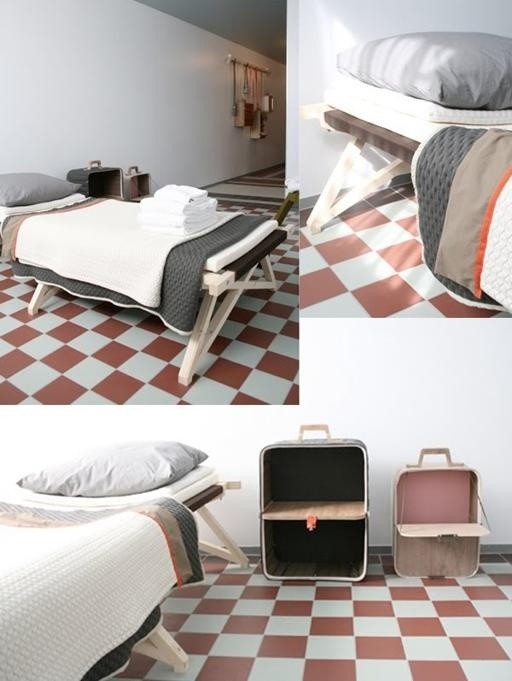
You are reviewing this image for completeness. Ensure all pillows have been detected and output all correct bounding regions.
[14,438,209,500]
[0,172,83,207]
[338,28,512,114]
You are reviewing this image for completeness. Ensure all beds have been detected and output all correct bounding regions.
[306,70,512,316]
[1,196,295,386]
[1,462,250,681]
